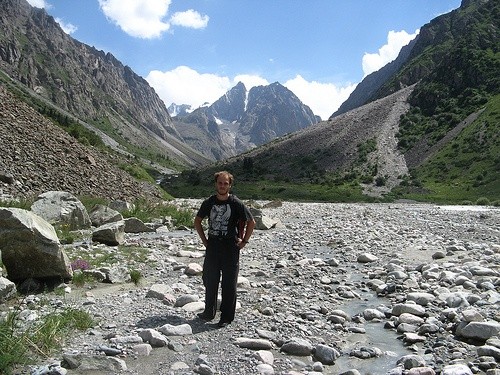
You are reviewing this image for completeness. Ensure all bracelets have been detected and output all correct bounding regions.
[242,238,249,244]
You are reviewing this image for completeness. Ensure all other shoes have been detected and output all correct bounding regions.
[218,318,232,324]
[198,313,214,320]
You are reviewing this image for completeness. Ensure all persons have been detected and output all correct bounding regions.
[193,170,254,328]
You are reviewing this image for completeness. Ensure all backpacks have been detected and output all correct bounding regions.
[213,193,245,240]
